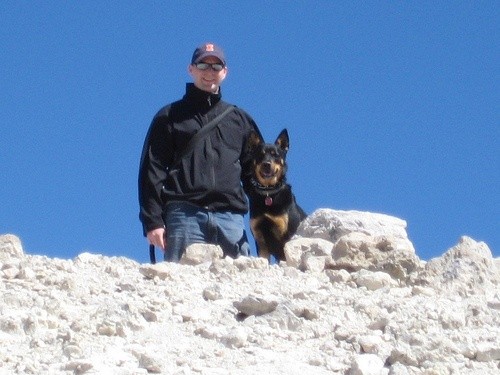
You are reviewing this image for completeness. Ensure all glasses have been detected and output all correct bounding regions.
[191,60,225,71]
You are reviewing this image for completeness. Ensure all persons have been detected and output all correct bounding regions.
[138,45,266,266]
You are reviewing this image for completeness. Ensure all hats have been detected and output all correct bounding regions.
[191,43,226,66]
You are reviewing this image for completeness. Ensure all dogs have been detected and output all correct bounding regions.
[247,128,307,263]
